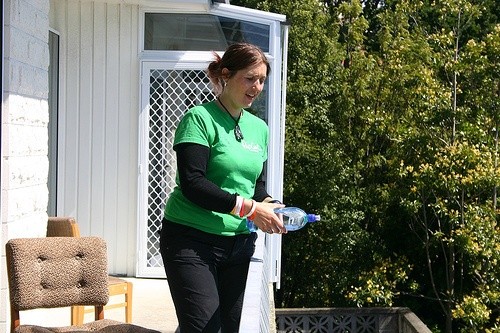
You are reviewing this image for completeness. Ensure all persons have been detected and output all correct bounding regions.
[160,43,288,333]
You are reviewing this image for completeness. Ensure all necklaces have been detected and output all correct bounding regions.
[217,95,244,140]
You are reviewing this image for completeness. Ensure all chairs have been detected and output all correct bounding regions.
[48,216,133,326]
[5,236,163,333]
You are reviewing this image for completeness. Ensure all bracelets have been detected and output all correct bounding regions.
[234,195,257,220]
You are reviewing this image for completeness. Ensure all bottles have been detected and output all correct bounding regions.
[247,207,321,232]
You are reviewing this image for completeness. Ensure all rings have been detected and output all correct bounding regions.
[267,228,271,232]
